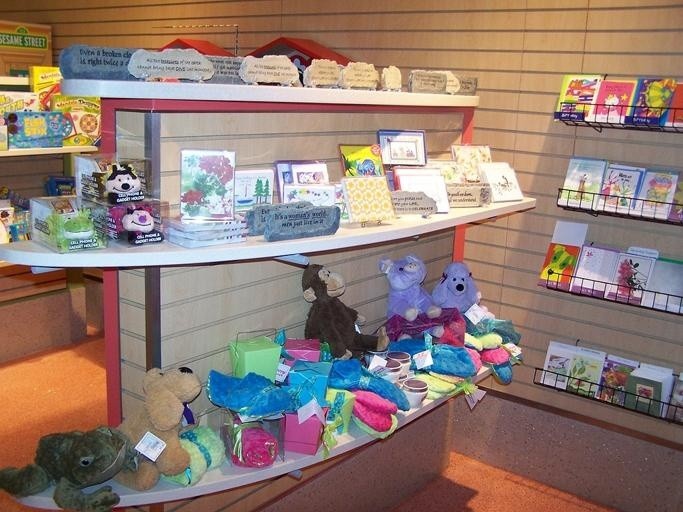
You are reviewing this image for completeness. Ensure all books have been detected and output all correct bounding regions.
[536,219,683,319]
[552,71,682,136]
[538,339,683,425]
[557,156,682,226]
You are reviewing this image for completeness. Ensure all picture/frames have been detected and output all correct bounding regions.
[376,127,427,167]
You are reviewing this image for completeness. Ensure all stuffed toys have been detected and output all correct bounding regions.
[0,423,144,511]
[376,251,446,343]
[301,264,389,365]
[431,259,482,314]
[62,163,154,241]
[109,363,203,493]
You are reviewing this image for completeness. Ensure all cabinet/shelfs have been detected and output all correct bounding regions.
[0,77,536,511]
[530,98,682,423]
